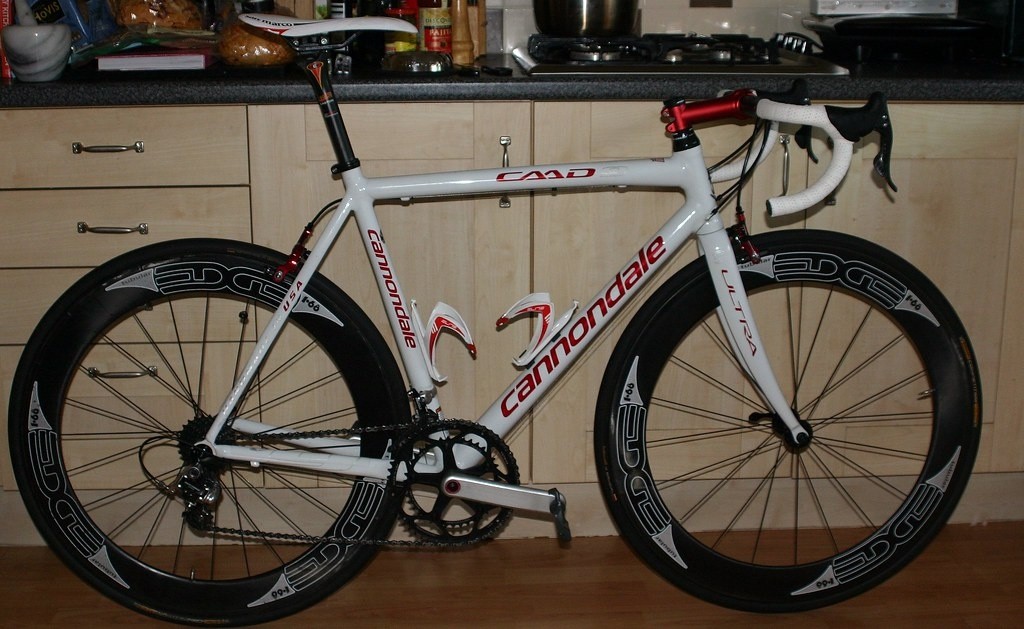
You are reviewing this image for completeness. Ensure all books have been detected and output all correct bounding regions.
[96,48,215,71]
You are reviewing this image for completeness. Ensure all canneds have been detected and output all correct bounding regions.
[418,7,453,56]
[383,8,417,57]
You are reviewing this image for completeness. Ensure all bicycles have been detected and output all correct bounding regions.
[7,8,985,629]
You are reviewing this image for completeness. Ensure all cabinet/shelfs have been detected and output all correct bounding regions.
[0,103,1023,549]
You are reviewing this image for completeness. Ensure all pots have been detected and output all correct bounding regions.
[532,0,640,39]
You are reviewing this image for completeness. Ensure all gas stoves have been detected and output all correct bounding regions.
[513,35,849,76]
